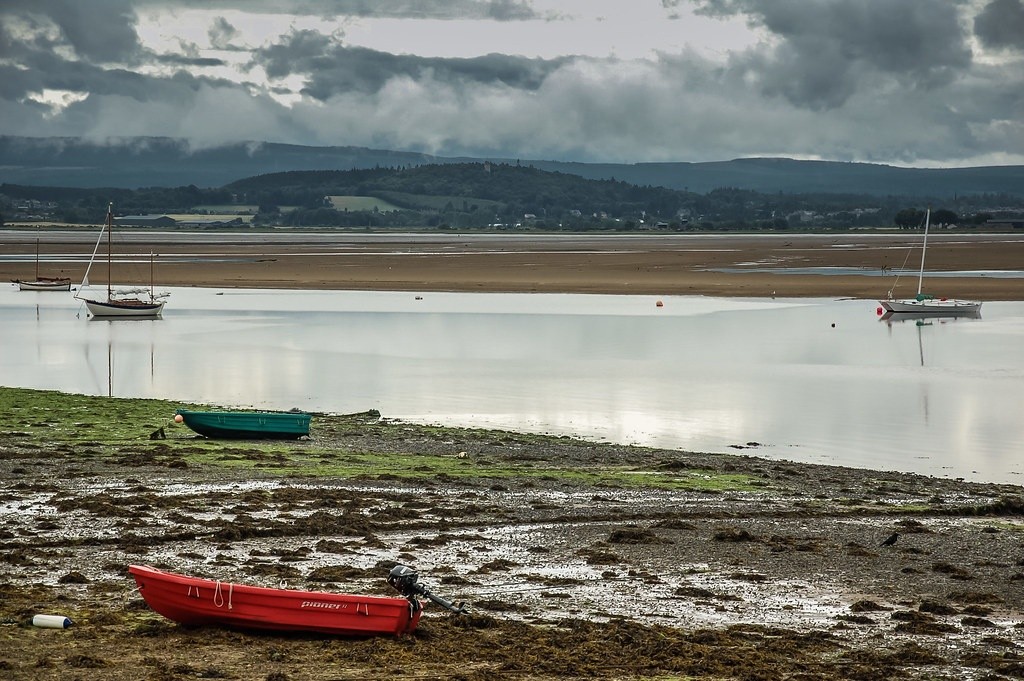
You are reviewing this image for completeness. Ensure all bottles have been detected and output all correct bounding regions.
[33,614,74,629]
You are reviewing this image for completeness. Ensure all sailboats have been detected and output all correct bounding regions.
[72,201,171,316]
[877,208,983,313]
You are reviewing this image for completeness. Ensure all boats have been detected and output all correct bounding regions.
[128,565,470,642]
[175,409,312,440]
[10,277,76,292]
